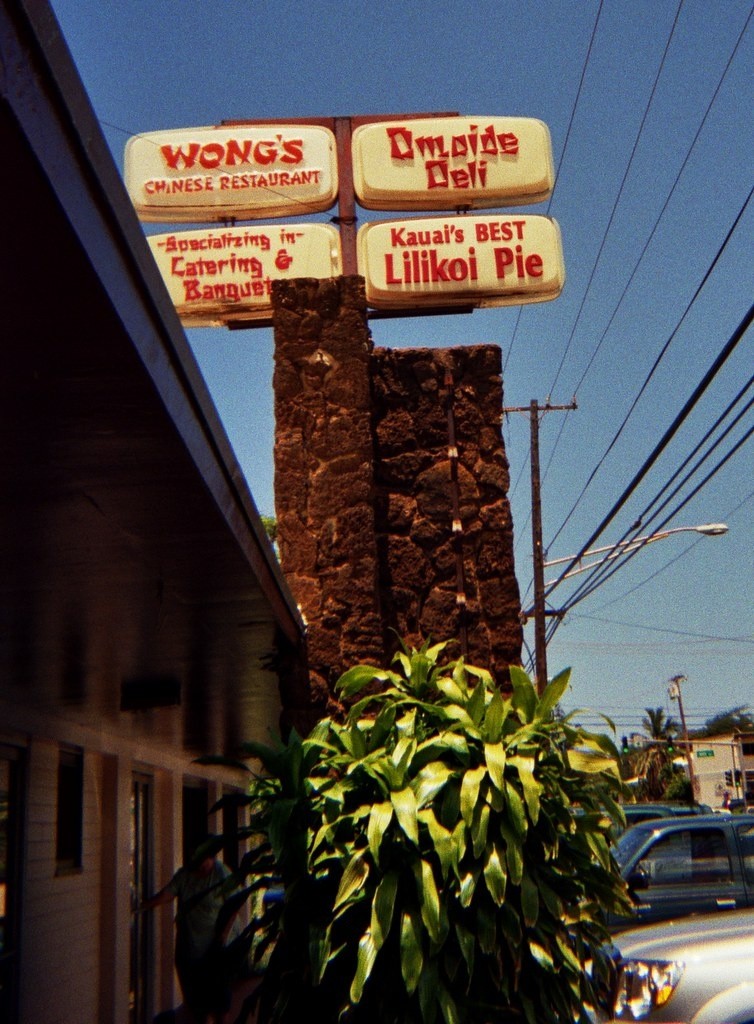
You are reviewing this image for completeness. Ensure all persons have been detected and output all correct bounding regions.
[132,833,243,1024]
[722,793,731,808]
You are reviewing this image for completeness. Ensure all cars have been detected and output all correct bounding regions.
[565,795,754,857]
[583,904,754,1024]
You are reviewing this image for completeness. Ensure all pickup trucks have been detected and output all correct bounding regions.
[590,814,754,936]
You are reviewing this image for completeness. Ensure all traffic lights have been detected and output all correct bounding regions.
[724,770,733,786]
[667,735,674,752]
[622,736,629,755]
[735,770,741,786]
